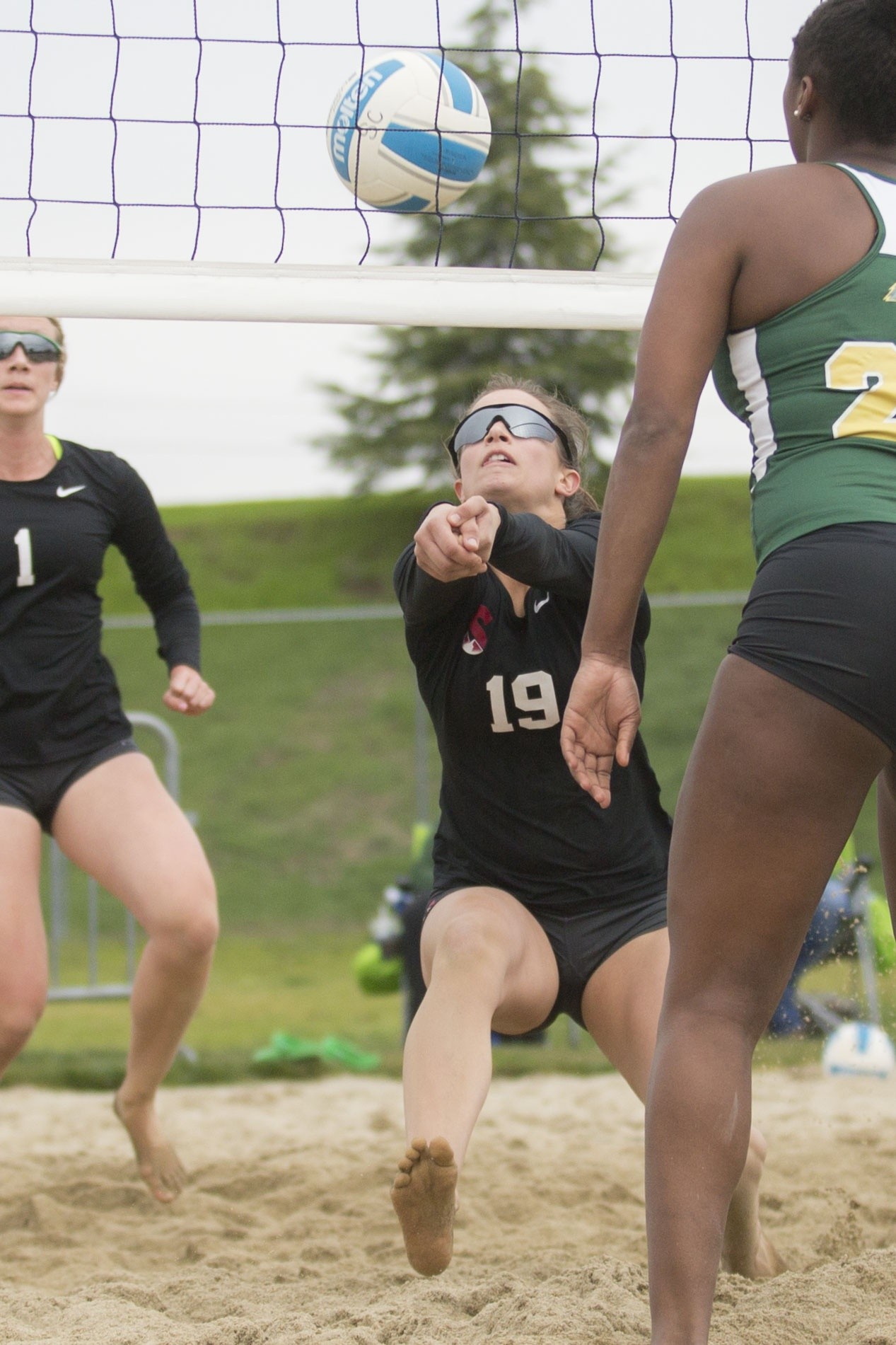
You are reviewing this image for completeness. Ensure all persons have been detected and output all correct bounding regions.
[558,0,896,1345]
[389,381,776,1282]
[0,313,219,1205]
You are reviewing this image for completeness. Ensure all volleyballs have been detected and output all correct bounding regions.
[821,1019,894,1077]
[326,48,490,214]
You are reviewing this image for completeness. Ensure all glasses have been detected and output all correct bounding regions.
[441,402,578,477]
[0,330,62,365]
